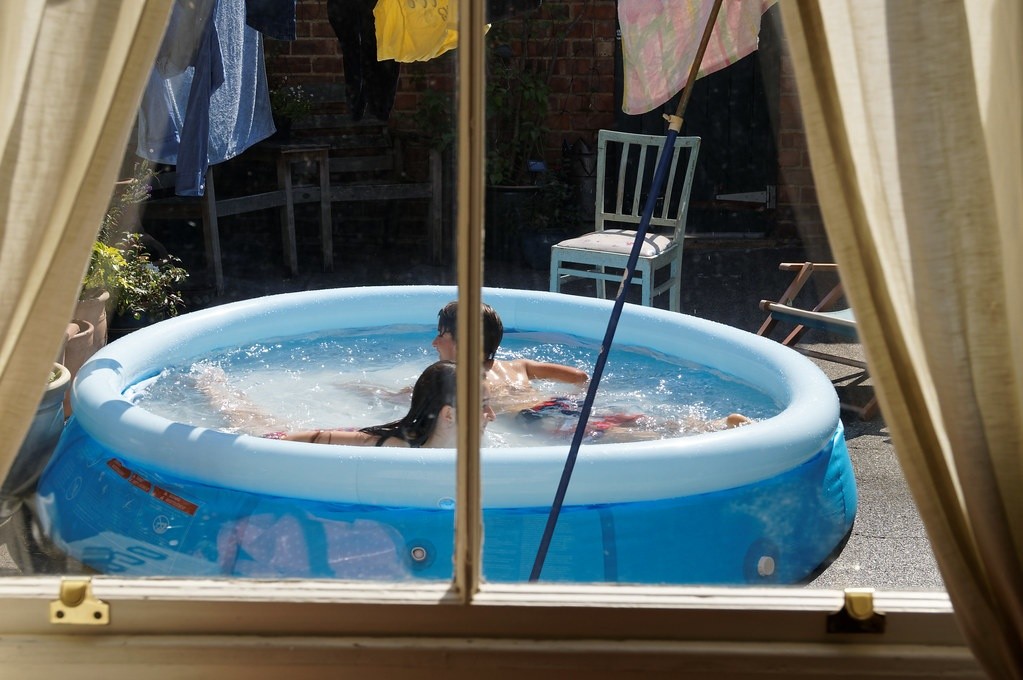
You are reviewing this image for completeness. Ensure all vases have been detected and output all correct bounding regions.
[1,361,72,499]
[261,119,293,144]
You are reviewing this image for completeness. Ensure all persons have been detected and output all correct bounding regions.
[432,300,747,444]
[285,362,495,447]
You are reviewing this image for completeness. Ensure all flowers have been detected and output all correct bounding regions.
[270,75,313,122]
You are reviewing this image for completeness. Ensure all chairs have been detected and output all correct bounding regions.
[550,130,702,311]
[140,79,444,297]
[757,264,879,418]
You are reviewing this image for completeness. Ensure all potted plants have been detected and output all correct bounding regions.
[516,168,585,271]
[80,160,190,345]
[61,319,95,418]
[388,0,589,261]
